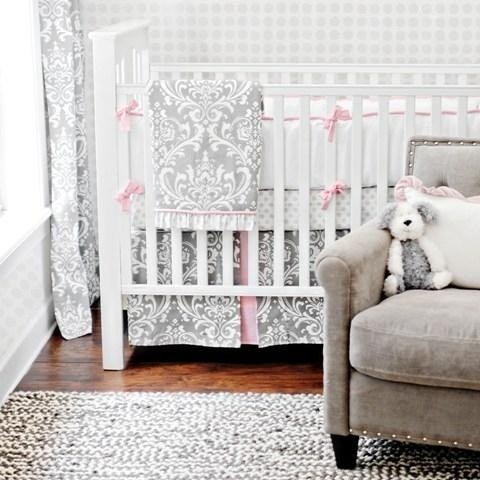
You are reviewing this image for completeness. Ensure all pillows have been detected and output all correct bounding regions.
[394,175,480,291]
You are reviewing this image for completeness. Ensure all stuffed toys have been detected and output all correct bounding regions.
[375,200,453,297]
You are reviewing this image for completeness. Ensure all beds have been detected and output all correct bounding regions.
[86,19,480,372]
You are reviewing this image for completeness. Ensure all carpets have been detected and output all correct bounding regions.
[0,390,480,480]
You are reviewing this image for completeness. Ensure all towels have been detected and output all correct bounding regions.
[143,78,265,232]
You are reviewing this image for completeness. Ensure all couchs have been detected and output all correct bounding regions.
[314,133,480,470]
